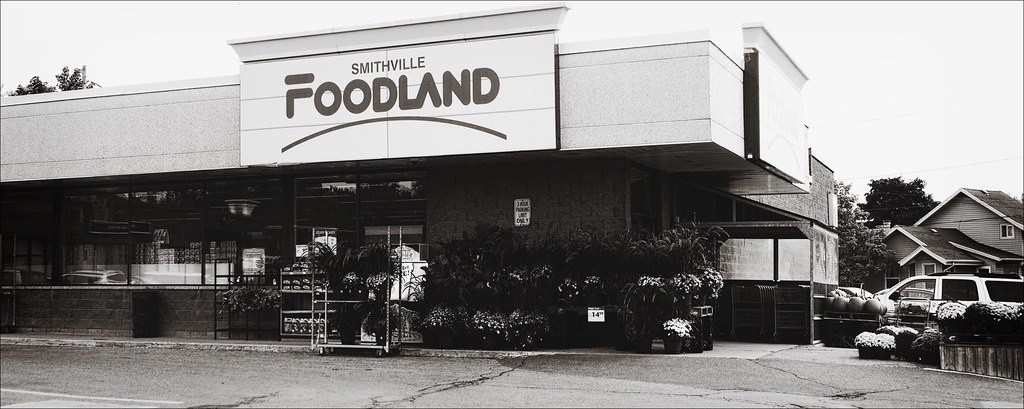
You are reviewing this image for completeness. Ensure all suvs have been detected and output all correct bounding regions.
[47,268,127,286]
[874,272,1024,313]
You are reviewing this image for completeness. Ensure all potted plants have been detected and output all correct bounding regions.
[402,212,730,351]
[357,237,410,300]
[965,302,992,342]
[363,304,402,346]
[327,303,374,344]
[300,240,362,298]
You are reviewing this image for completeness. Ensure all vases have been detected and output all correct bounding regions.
[888,335,914,354]
[942,319,965,333]
[662,299,673,313]
[543,316,575,348]
[556,289,577,307]
[348,290,362,300]
[920,350,940,366]
[373,289,387,301]
[858,346,892,360]
[420,328,453,348]
[677,293,692,306]
[582,289,604,307]
[692,292,707,306]
[663,335,683,354]
[485,327,528,351]
[994,320,1024,343]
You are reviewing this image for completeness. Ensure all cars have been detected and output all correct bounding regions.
[876,286,956,300]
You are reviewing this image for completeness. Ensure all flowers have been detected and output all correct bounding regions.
[854,331,897,352]
[408,303,471,335]
[910,328,946,352]
[667,271,703,294]
[580,274,607,297]
[876,325,920,340]
[558,277,579,298]
[472,308,553,349]
[214,285,284,313]
[937,302,967,320]
[637,274,674,296]
[550,306,564,318]
[365,271,395,301]
[988,302,1024,322]
[339,270,365,293]
[693,264,724,298]
[662,317,695,339]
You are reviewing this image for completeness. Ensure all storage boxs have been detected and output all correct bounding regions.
[283,280,290,284]
[309,261,318,267]
[314,285,323,290]
[67,241,237,270]
[302,268,309,272]
[309,318,317,334]
[303,285,311,290]
[284,267,291,271]
[294,286,301,290]
[317,318,325,334]
[293,268,301,273]
[293,280,301,285]
[283,285,290,290]
[284,318,292,334]
[300,318,308,334]
[292,262,300,268]
[314,279,322,284]
[303,278,311,284]
[292,318,301,334]
[300,262,309,268]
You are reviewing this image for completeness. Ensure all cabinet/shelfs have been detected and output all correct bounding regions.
[280,267,336,336]
[312,225,402,358]
